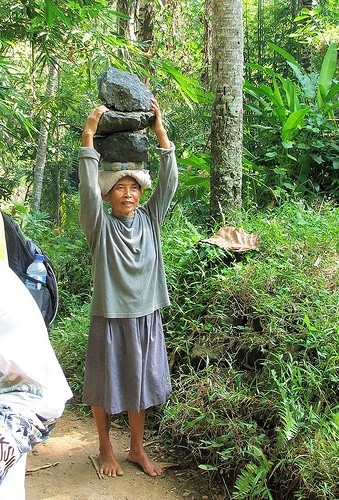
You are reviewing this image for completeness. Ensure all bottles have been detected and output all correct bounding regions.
[25,253,47,290]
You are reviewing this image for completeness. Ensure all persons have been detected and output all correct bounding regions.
[0,262,74,500]
[77,92,179,479]
[0,210,58,327]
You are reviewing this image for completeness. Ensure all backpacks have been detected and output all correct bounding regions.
[0,210,58,330]
